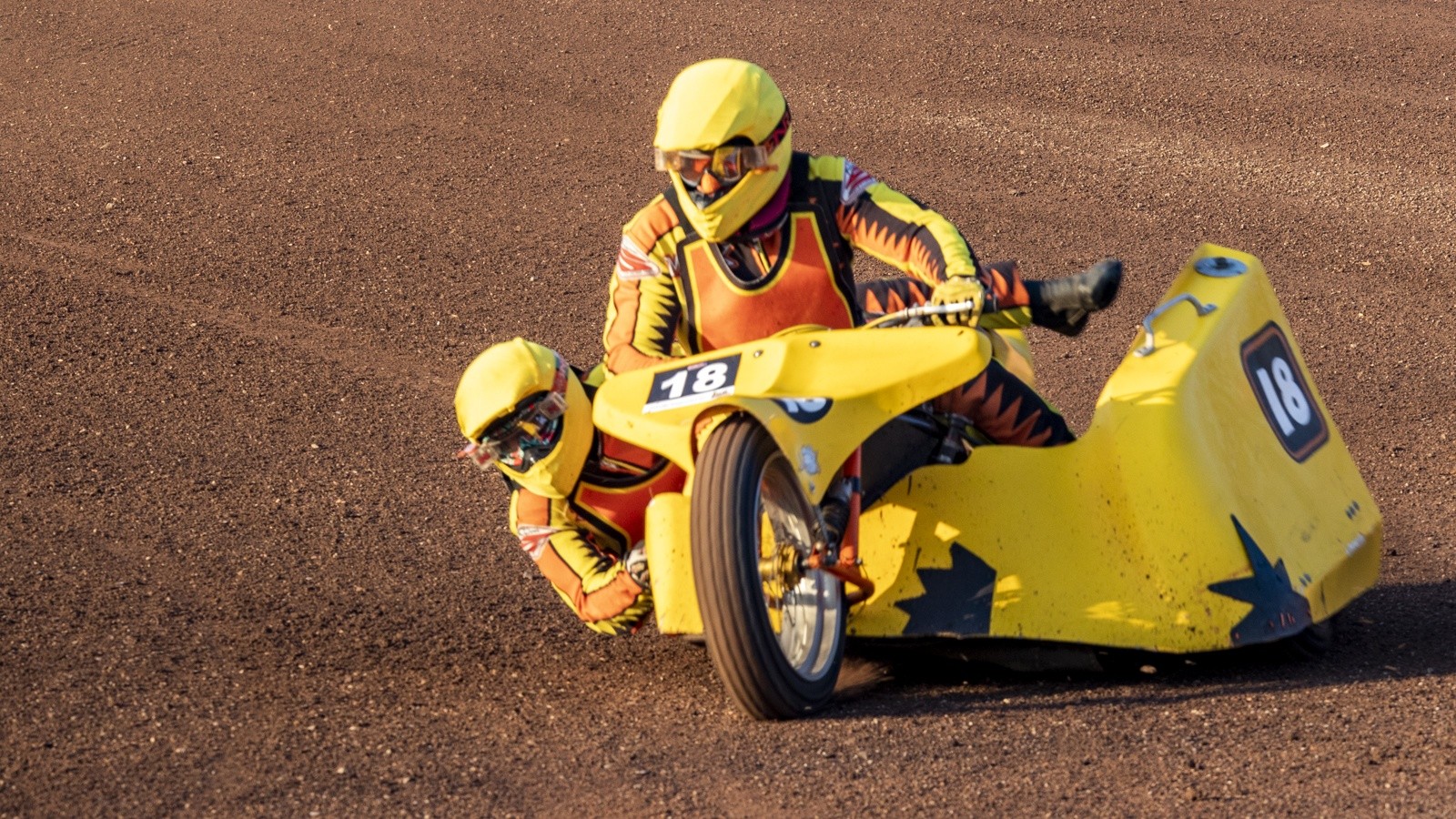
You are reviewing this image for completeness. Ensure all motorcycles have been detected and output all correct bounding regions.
[593,244,1383,721]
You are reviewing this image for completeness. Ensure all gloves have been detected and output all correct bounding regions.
[930,275,985,328]
[625,540,651,590]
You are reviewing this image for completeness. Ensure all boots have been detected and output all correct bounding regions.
[1023,260,1121,336]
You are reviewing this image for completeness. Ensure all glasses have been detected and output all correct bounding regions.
[674,147,751,187]
[478,400,563,473]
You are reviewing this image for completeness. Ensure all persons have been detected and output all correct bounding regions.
[451,257,1133,643]
[598,56,1079,448]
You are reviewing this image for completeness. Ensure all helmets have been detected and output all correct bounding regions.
[654,58,792,243]
[454,337,594,499]
